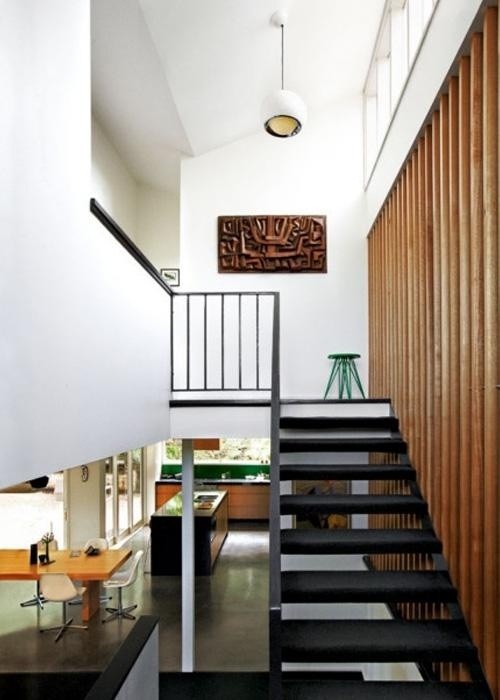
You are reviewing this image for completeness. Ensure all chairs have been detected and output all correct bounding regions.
[21,539,144,642]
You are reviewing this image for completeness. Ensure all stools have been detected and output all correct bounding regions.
[323,350,367,400]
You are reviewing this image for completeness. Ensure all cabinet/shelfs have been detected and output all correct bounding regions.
[156,485,269,518]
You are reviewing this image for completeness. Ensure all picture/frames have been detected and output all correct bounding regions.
[291,478,352,531]
[160,268,180,287]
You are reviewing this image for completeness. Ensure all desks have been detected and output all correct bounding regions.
[0,546,133,622]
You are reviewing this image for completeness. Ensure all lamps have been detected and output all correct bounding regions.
[260,9,310,139]
